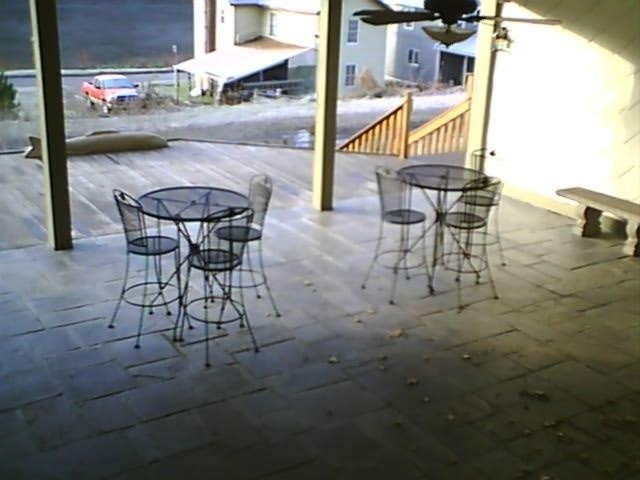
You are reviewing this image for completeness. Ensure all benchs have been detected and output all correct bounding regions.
[556,186,640,256]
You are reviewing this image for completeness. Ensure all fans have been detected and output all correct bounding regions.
[353,0,563,49]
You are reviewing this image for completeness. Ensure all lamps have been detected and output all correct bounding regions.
[421,20,476,48]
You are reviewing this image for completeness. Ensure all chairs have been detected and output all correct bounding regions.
[360,148,508,313]
[108,171,280,370]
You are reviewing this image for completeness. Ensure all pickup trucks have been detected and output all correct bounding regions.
[80,74,141,114]
